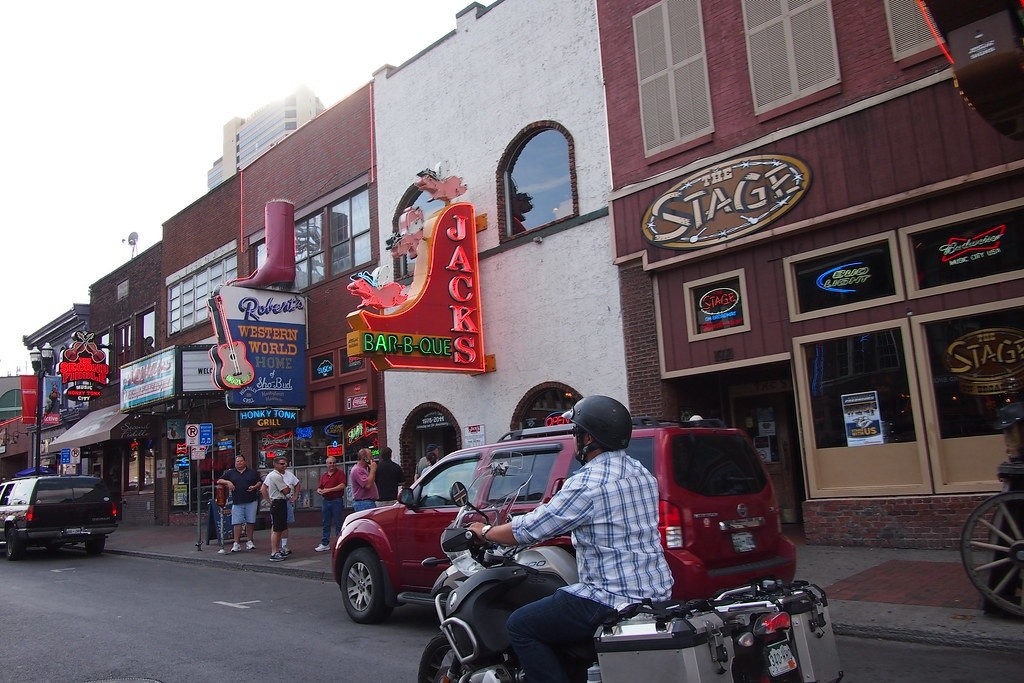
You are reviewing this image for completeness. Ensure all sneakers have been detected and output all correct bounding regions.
[314,544,331,552]
[231,542,241,552]
[245,540,256,550]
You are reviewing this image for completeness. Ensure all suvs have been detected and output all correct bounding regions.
[330,418,799,625]
[0,476,119,561]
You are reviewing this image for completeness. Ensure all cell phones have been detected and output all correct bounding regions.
[362,456,371,464]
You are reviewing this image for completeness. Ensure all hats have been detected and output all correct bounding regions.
[426,444,440,452]
[561,394,633,451]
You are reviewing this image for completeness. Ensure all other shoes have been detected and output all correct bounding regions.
[269,552,289,562]
[279,547,291,555]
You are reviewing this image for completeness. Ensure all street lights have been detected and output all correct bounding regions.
[29,343,54,475]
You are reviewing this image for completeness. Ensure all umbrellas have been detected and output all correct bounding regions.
[16,466,56,477]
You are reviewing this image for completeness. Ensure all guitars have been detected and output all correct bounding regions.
[206,294,256,391]
[63,332,106,364]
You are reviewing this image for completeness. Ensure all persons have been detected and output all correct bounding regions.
[315,456,348,552]
[413,444,442,483]
[375,447,407,508]
[468,395,674,683]
[350,448,379,513]
[260,456,301,562]
[217,455,263,551]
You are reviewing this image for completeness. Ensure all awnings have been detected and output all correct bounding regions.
[48,404,163,452]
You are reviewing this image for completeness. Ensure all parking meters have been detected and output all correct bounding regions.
[216,484,228,554]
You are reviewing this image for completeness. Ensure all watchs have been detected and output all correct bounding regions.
[481,524,493,543]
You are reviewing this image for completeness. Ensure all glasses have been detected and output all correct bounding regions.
[571,426,578,437]
[277,463,285,465]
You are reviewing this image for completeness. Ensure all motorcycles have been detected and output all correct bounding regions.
[420,449,844,683]
